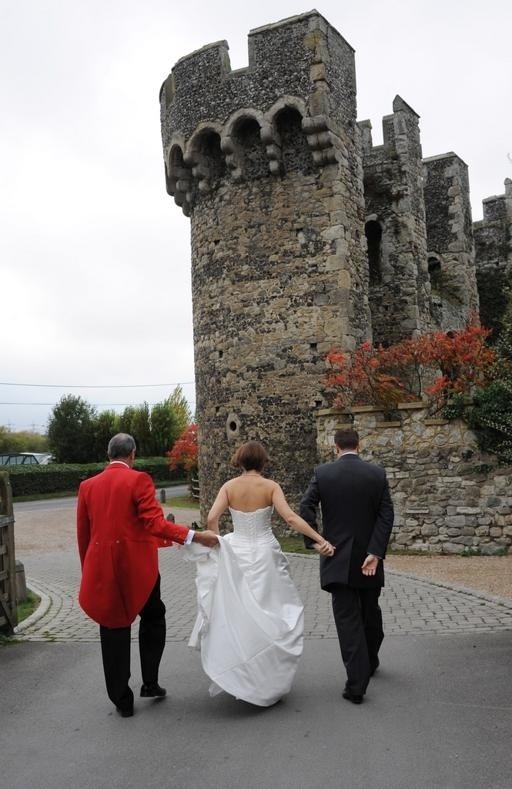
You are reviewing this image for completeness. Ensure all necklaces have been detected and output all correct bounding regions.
[242,474,261,476]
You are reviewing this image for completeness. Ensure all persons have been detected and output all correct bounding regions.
[77,433,218,718]
[301,429,394,703]
[187,440,336,706]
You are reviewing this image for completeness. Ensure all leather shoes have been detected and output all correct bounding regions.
[115,704,134,718]
[139,683,167,698]
[341,681,367,704]
